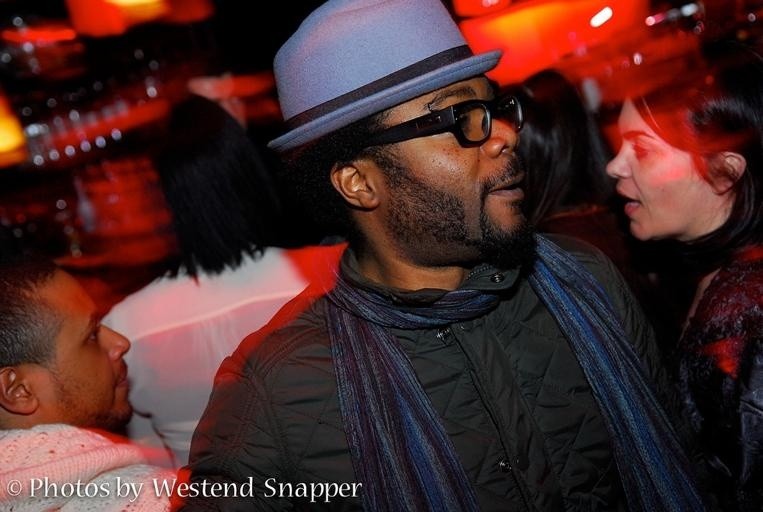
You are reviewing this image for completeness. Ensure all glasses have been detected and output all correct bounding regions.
[349,92,525,168]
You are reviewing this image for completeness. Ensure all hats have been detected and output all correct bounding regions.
[268,0,502,152]
